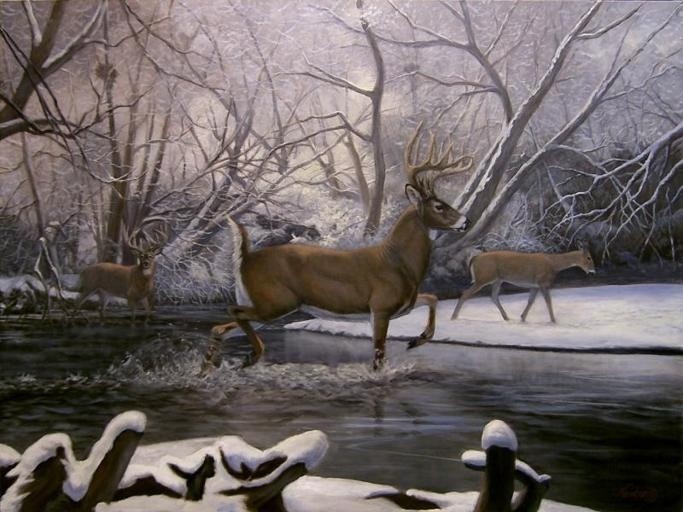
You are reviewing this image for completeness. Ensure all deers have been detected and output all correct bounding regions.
[200,120,477,372]
[451,240,596,324]
[64,228,168,330]
